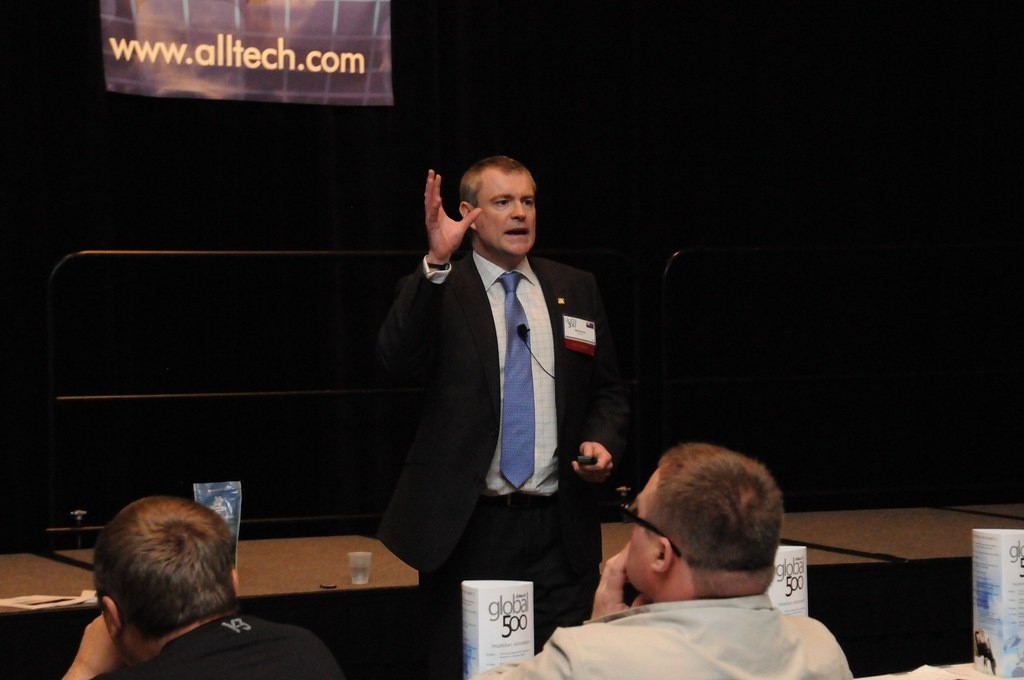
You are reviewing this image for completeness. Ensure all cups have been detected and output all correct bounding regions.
[191,482,243,585]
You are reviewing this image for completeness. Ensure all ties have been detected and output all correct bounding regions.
[495,270,535,490]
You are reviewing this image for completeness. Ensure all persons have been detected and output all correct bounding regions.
[59,495,344,680]
[371,156,633,680]
[466,442,856,680]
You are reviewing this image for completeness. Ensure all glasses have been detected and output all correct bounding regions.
[619,503,682,558]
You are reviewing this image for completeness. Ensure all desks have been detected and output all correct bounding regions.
[592,503,1024,680]
[0,534,417,680]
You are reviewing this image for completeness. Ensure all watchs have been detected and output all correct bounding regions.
[428,262,449,270]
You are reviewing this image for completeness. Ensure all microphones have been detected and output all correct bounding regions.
[517,324,528,342]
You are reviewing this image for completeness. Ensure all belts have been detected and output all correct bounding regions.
[482,491,531,507]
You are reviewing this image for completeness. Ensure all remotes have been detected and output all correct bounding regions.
[578,455,597,466]
